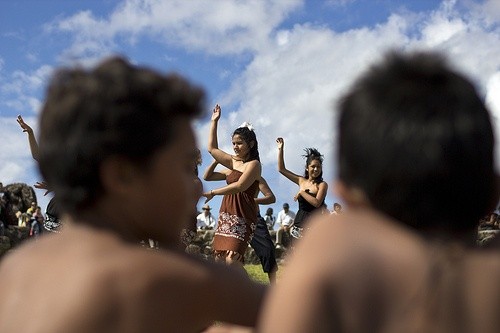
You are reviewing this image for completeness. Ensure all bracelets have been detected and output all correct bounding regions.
[210,190,215,196]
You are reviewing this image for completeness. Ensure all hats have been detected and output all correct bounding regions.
[283,203,289,208]
[202,205,212,210]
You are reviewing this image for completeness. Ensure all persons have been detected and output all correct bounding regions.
[275,137,331,256]
[201,103,262,283]
[0,47,500,333]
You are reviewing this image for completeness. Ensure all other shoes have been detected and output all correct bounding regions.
[275,244,281,249]
